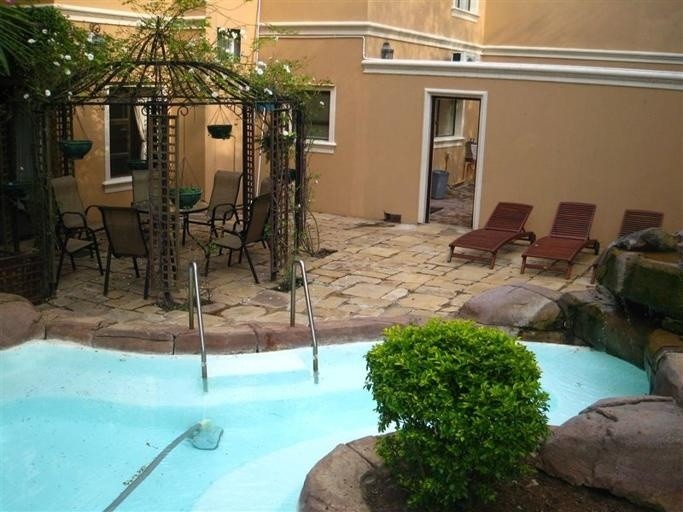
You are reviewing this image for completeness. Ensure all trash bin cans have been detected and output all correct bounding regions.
[431,170,449,199]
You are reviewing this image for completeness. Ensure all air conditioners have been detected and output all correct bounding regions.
[449,52,475,63]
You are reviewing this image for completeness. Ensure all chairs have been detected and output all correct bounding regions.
[50,166,281,300]
[447,198,662,281]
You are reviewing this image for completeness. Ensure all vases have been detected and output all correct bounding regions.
[62,140,92,159]
[207,124,231,139]
